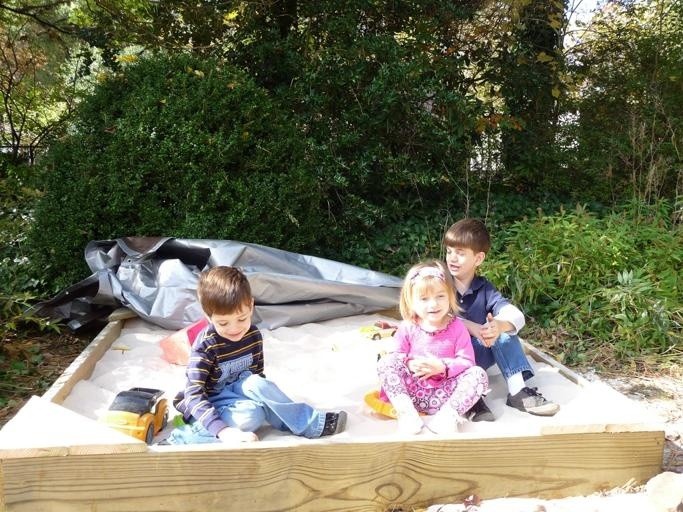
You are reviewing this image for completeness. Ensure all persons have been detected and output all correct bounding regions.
[440,217,561,424]
[173,265,347,445]
[374,258,489,437]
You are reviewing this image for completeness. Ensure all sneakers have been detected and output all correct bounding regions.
[425,408,459,433]
[464,397,495,421]
[399,414,423,435]
[506,386,560,416]
[321,411,346,435]
